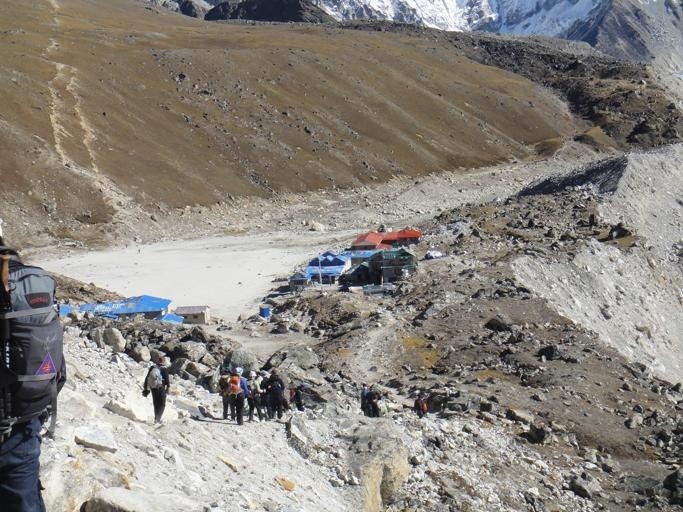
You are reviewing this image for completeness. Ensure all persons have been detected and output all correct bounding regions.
[413,394,427,418]
[0,236,65,511]
[360,381,387,417]
[141,355,172,428]
[218,362,302,424]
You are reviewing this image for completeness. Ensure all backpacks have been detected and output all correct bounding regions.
[0,247,65,428]
[246,382,255,399]
[147,365,163,388]
[229,376,241,394]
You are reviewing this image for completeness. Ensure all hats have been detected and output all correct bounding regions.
[236,367,243,374]
[248,371,256,377]
